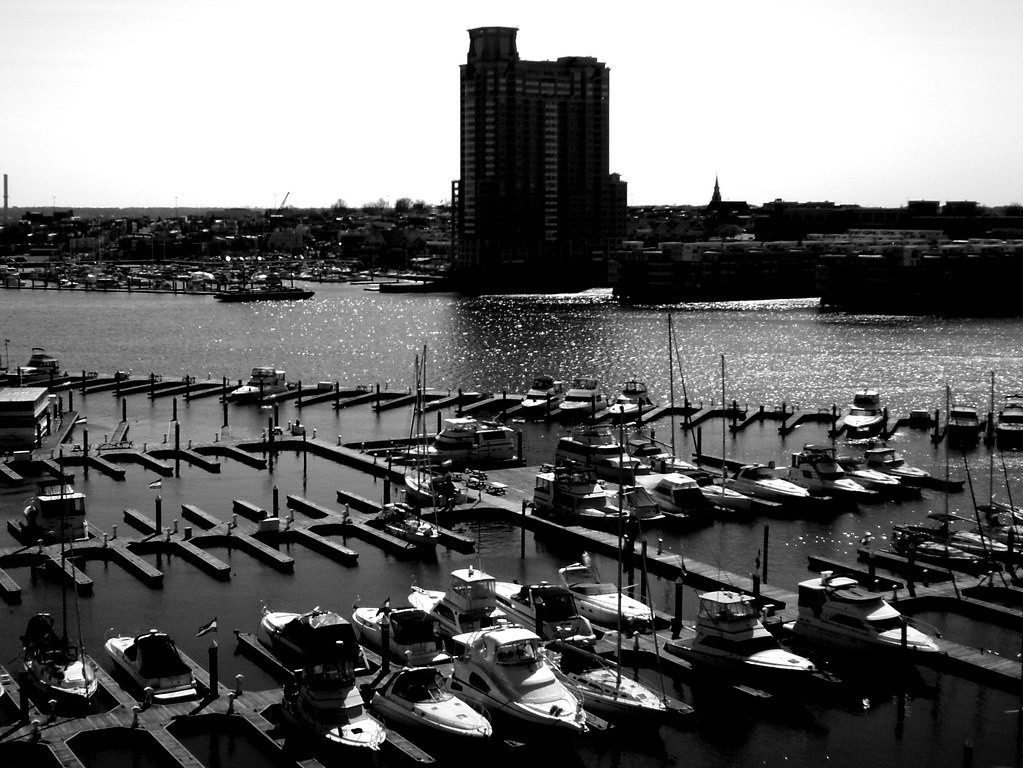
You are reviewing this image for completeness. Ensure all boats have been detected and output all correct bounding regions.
[949,402,986,445]
[796,569,940,656]
[6,348,61,380]
[521,376,657,417]
[349,551,656,667]
[997,393,1023,444]
[371,623,666,738]
[231,369,287,397]
[260,600,370,675]
[282,660,387,753]
[533,305,931,527]
[103,628,198,703]
[844,388,882,439]
[23,483,89,542]
[215,286,316,301]
[663,534,817,683]
[398,412,515,461]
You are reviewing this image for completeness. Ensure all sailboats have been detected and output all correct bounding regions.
[404,353,468,505]
[894,371,1023,572]
[379,345,441,545]
[20,448,98,712]
